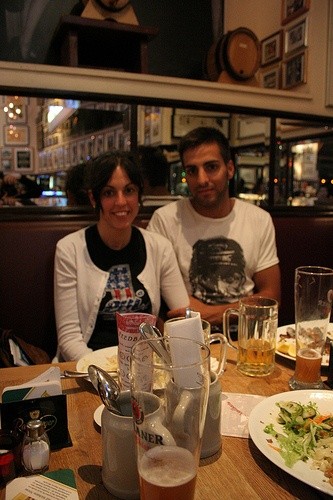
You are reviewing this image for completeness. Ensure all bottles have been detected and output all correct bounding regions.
[22,420,50,475]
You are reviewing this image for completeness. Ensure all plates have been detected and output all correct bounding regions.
[93,404,105,427]
[249,390,333,497]
[275,322,333,365]
[77,345,171,390]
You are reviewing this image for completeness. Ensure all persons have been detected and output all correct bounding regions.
[65,163,94,206]
[147,126,280,345]
[137,146,170,195]
[54,150,189,361]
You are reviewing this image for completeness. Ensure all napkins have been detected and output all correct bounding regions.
[219,391,266,439]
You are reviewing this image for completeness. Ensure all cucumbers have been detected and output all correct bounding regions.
[301,406,316,419]
[278,402,303,415]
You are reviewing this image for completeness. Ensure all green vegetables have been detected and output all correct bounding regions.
[263,401,327,468]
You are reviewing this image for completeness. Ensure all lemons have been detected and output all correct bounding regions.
[277,339,302,358]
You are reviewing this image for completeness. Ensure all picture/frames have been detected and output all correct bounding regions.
[282,15,309,54]
[281,48,308,89]
[260,29,283,67]
[261,68,280,91]
[170,108,231,143]
[223,27,262,80]
[279,0,311,25]
[237,118,270,140]
[14,147,34,173]
[3,95,29,125]
[2,124,30,147]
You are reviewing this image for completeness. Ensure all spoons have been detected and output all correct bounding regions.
[89,365,123,415]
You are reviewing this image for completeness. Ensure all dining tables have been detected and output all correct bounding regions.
[0,328,333,500]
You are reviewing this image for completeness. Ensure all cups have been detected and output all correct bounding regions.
[166,370,223,459]
[162,317,224,376]
[131,338,212,500]
[117,367,153,397]
[289,266,333,390]
[223,296,279,377]
[101,390,176,499]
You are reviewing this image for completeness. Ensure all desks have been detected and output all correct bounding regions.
[59,14,158,75]
